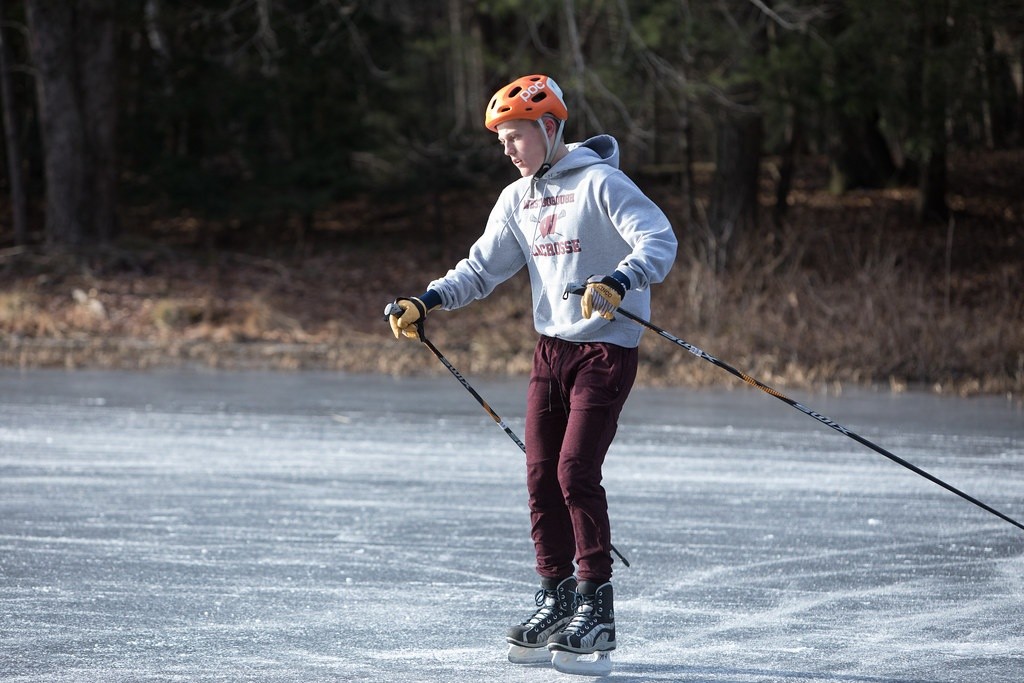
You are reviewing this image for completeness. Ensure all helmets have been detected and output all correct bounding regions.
[485,74,568,131]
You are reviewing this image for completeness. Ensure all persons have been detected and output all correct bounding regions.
[389,74,679,676]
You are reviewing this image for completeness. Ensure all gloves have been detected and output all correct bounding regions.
[388,298,426,340]
[581,274,627,319]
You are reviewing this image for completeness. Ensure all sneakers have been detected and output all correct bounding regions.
[506,577,579,665]
[548,582,617,676]
